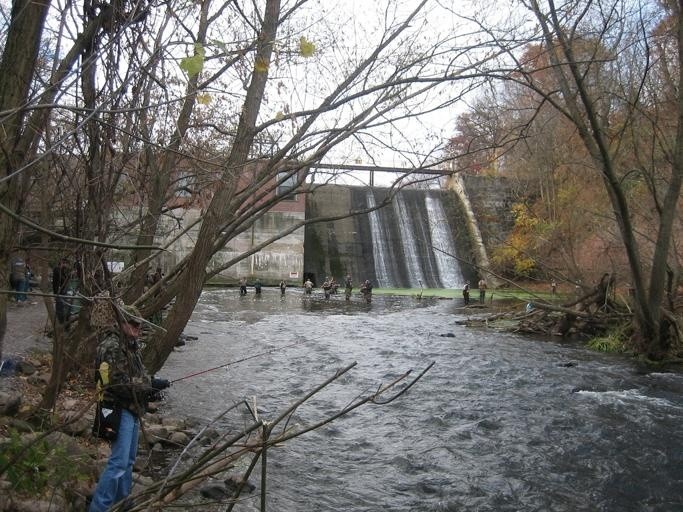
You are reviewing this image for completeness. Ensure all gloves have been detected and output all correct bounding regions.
[152,379,169,389]
[144,389,163,402]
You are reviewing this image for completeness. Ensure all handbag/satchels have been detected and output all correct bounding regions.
[92,401,123,441]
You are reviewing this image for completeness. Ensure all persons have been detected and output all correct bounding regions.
[90,266,171,295]
[87,304,174,511]
[344,274,354,300]
[478,277,491,304]
[254,278,263,297]
[52,258,70,322]
[462,279,470,306]
[238,276,248,296]
[13,257,34,303]
[360,279,374,304]
[303,278,314,295]
[321,276,340,301]
[280,279,286,294]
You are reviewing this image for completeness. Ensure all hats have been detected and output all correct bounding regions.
[117,305,144,324]
